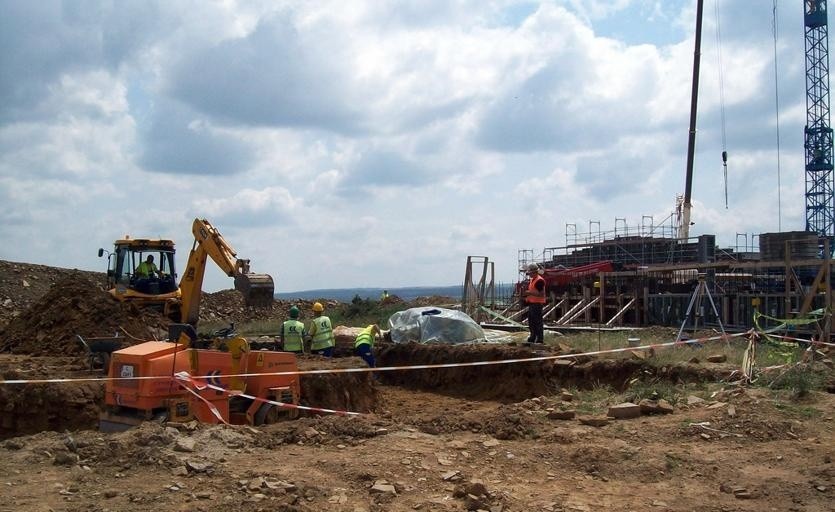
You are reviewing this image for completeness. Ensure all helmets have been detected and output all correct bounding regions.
[290,306,299,314]
[312,302,324,311]
[526,264,539,275]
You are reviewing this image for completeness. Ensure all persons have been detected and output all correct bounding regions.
[280,306,307,353]
[308,302,335,356]
[523,265,547,343]
[134,254,164,279]
[352,294,362,303]
[354,323,384,385]
[380,290,389,300]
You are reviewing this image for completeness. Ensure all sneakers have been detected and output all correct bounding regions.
[528,338,543,343]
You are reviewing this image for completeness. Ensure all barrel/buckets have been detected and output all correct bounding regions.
[628,338,641,347]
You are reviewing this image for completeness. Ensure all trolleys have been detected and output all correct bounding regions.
[65,324,124,374]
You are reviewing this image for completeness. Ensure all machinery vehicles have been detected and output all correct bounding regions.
[101,308,306,437]
[96,219,281,337]
